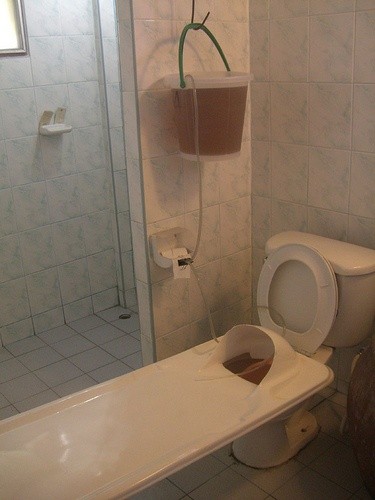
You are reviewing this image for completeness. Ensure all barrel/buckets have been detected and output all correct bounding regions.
[163,23,254,161]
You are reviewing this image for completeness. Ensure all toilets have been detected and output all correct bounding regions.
[229,229,374,468]
